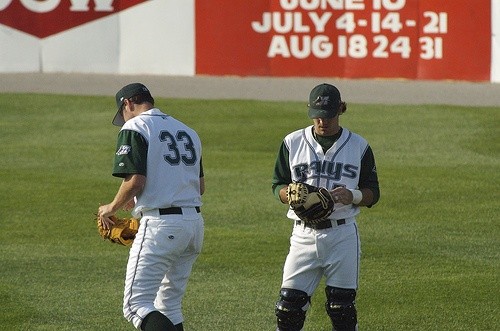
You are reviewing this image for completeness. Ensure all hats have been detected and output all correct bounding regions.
[310,84,341,119]
[112,83,151,127]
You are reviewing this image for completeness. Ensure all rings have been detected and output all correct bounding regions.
[335,195,338,201]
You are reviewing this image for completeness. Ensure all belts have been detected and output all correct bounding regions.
[295,219,346,230]
[141,206,200,216]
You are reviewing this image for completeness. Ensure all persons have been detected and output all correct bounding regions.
[271,83,381,331]
[97,83,206,331]
